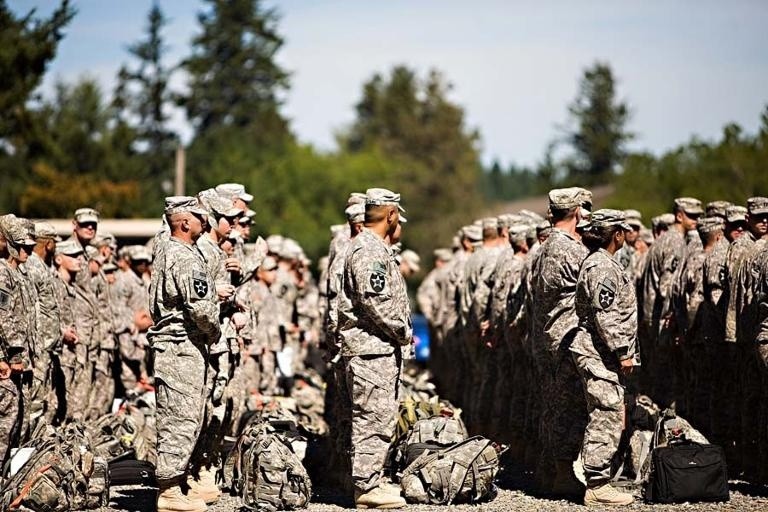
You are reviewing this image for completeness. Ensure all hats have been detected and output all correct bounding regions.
[433,186,768,262]
[165,183,257,228]
[119,246,152,262]
[403,249,421,271]
[331,224,344,240]
[346,188,407,224]
[229,229,241,242]
[0,208,116,260]
[244,233,310,268]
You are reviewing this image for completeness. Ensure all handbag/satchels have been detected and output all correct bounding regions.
[645,435,732,503]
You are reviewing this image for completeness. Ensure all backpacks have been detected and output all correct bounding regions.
[0,399,157,512]
[398,363,506,506]
[231,362,333,511]
[622,394,715,481]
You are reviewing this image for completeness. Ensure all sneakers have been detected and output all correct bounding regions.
[351,479,407,510]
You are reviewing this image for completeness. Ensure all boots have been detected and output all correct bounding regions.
[157,478,210,511]
[510,437,582,496]
[582,478,637,507]
[180,472,218,502]
